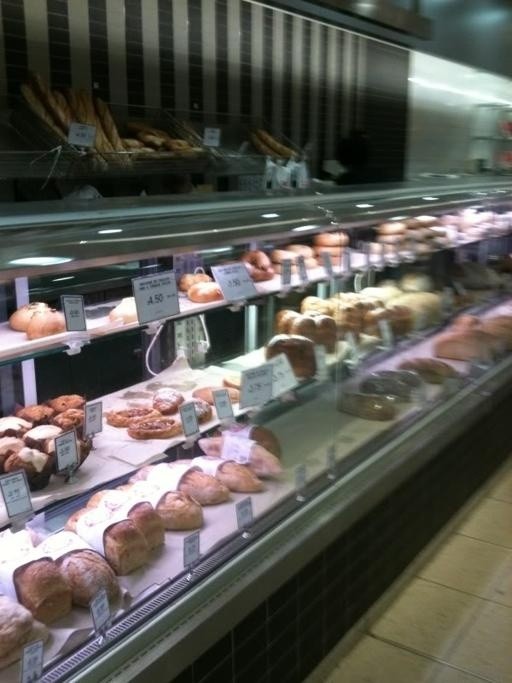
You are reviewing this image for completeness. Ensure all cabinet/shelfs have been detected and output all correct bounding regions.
[1,174,512,682]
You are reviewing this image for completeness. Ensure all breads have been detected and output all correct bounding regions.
[42,87,302,170]
[0,207,512,671]
